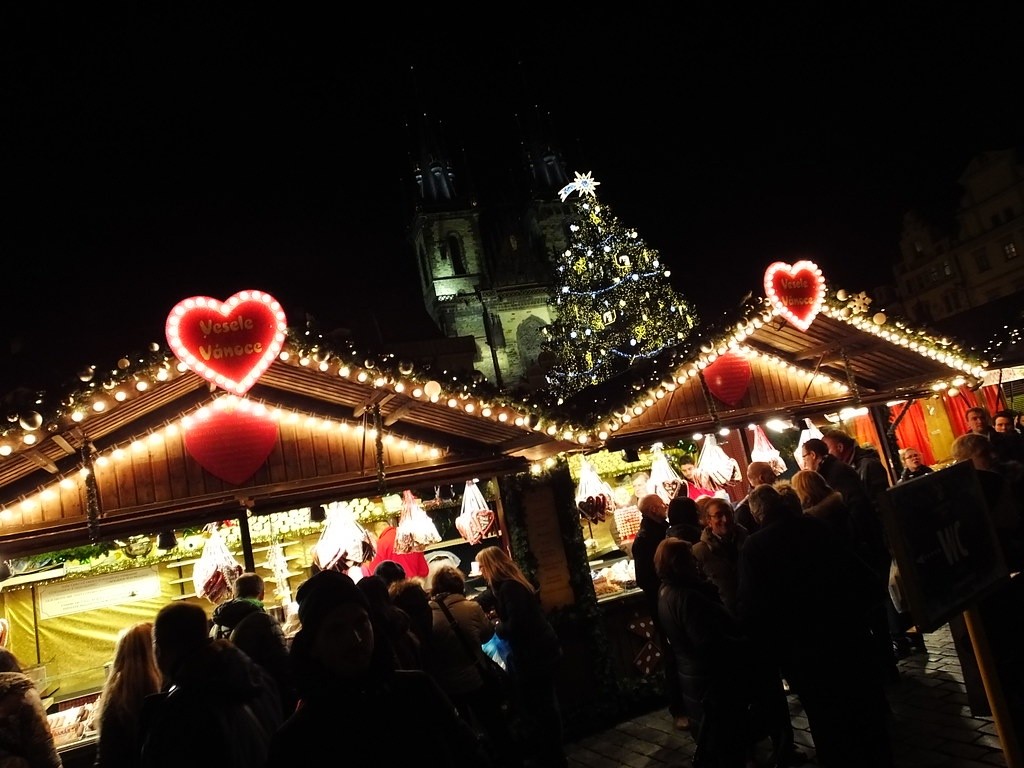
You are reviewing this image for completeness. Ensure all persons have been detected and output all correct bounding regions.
[0,619,63,768]
[631,406,1024,768]
[96,546,571,768]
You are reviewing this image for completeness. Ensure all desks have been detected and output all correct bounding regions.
[424,530,501,580]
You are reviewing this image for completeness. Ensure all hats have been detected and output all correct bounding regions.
[296,569,373,640]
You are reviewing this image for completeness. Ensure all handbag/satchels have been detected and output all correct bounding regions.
[478,660,511,697]
